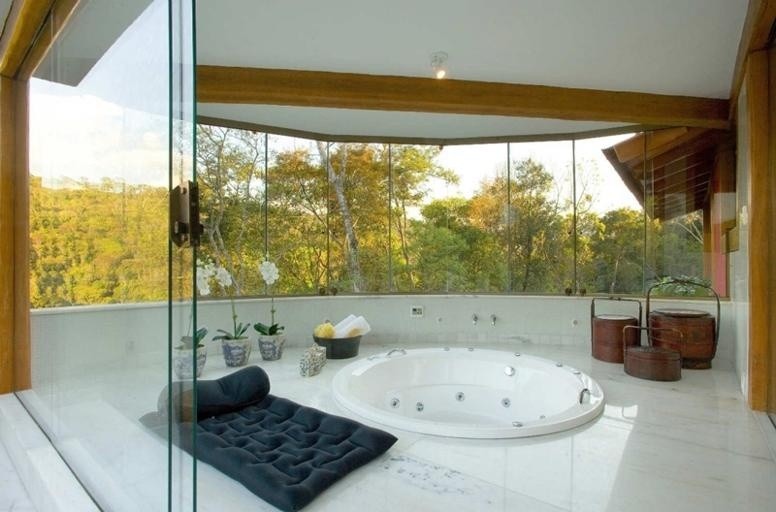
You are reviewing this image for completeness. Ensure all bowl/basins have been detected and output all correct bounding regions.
[311,333,362,359]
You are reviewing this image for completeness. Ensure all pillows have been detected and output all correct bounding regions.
[158,366,271,421]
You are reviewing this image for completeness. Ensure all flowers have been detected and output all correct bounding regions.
[254,261,285,335]
[181,266,211,348]
[212,267,250,340]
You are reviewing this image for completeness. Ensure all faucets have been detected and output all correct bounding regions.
[385,348,407,356]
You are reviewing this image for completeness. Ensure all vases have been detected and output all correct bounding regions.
[222,336,252,367]
[258,334,286,361]
[174,345,206,379]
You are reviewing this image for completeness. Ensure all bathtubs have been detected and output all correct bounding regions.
[332,346,605,440]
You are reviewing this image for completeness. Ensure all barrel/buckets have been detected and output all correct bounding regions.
[589,296,642,365]
[646,278,720,369]
